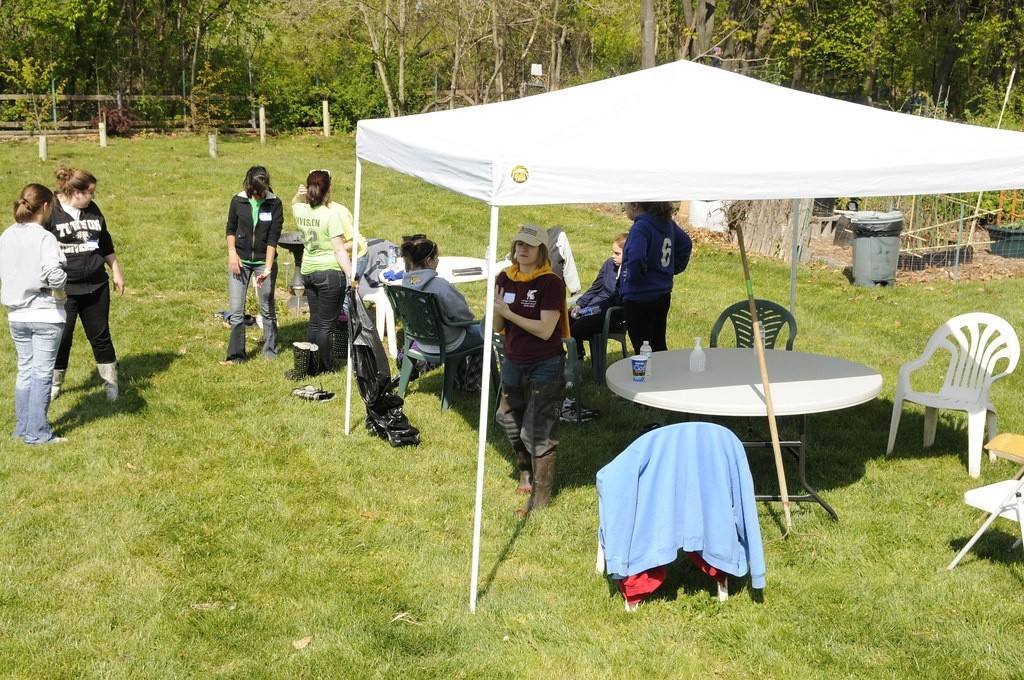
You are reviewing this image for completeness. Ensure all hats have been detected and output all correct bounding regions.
[515,223,549,249]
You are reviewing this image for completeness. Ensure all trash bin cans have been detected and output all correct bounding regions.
[843,208,905,291]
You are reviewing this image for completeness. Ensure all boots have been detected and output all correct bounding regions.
[513,445,533,495]
[49,370,66,402]
[284,343,309,380]
[516,451,557,516]
[308,349,319,376]
[95,361,119,402]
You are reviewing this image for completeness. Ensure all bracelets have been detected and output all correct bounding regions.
[263,269,271,274]
[228,248,236,251]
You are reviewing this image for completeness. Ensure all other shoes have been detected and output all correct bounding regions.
[53,436,67,441]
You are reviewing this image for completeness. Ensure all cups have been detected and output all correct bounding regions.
[631,355,647,382]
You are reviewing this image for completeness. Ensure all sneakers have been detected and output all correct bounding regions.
[561,397,601,425]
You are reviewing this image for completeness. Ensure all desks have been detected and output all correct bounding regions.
[379,255,489,286]
[605,346,882,524]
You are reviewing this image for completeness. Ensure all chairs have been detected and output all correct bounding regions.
[382,284,482,411]
[976,432,1024,520]
[886,311,1021,478]
[946,476,1024,572]
[358,275,398,357]
[710,299,797,351]
[480,312,581,424]
[566,294,627,386]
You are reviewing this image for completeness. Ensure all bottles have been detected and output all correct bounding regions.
[639,341,652,375]
[754,320,766,357]
[575,305,602,317]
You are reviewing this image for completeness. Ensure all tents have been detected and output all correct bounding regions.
[346,58,1024,614]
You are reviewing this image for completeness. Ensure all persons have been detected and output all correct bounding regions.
[620,202,692,355]
[558,367,601,422]
[218,162,285,367]
[290,169,369,373]
[492,223,572,515]
[0,183,69,445]
[377,234,485,392]
[41,164,125,403]
[568,233,629,362]
